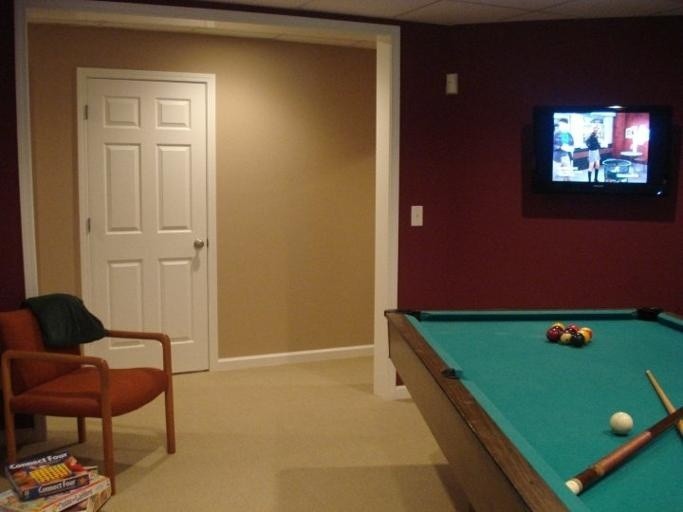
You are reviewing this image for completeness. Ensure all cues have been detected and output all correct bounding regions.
[565,405,683,496]
[646,370,683,435]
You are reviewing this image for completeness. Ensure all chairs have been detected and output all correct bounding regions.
[0,308,176,496]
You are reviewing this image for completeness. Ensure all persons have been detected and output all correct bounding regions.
[552,118,576,182]
[582,125,601,182]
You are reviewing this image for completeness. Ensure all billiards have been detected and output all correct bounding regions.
[610,412,633,434]
[546,321,593,347]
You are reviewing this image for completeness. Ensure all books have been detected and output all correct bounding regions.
[0,468,111,511]
[6,449,89,503]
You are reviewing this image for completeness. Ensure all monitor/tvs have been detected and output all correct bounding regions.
[532,103,673,199]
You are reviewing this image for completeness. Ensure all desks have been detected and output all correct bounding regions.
[384,304,682,512]
